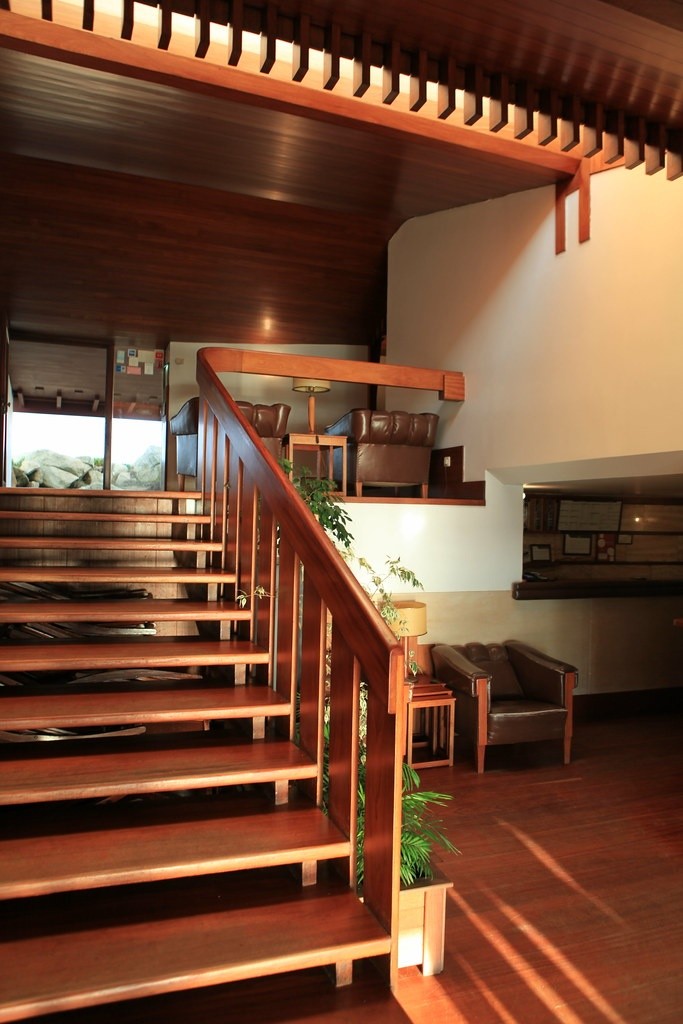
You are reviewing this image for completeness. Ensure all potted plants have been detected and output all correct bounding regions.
[319,723,454,976]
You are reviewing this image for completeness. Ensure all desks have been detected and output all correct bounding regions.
[283,434,348,497]
[402,677,458,771]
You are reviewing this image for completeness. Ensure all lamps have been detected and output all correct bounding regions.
[387,600,427,682]
[291,377,330,434]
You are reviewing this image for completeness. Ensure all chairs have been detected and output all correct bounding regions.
[431,639,579,774]
[325,408,439,497]
[169,397,291,492]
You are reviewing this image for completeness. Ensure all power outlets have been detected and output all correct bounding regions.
[444,457,451,467]
